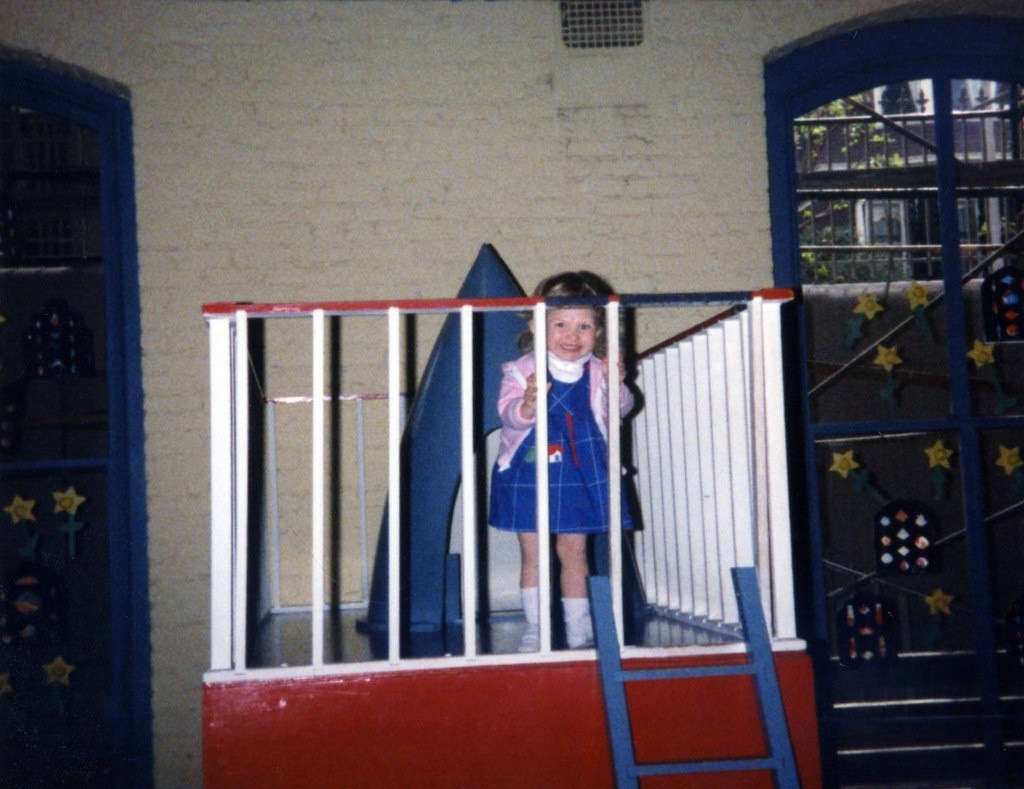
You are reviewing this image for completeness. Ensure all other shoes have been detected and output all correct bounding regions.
[572,639,597,649]
[517,623,540,654]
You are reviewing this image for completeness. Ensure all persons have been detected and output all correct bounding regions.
[490,270,638,652]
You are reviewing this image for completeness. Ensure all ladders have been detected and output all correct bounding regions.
[586,568,802,788]
